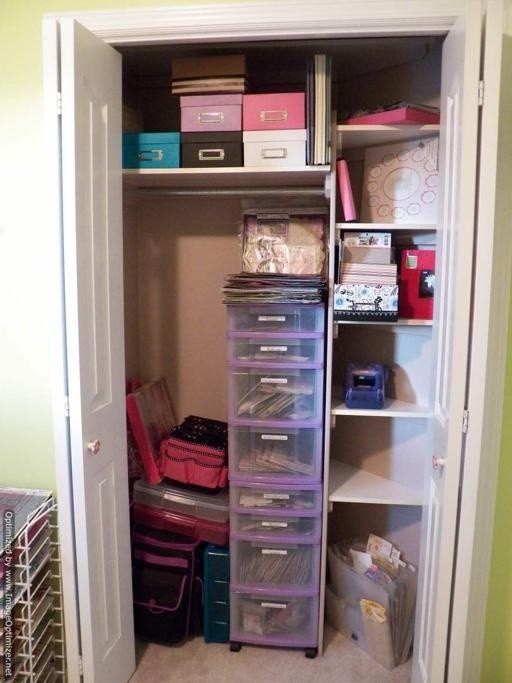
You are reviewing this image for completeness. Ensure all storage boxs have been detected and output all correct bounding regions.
[133,477,229,525]
[203,544,229,644]
[179,132,242,167]
[242,129,307,166]
[123,132,180,168]
[334,283,398,322]
[170,55,254,94]
[243,93,306,131]
[179,95,242,131]
[131,504,230,547]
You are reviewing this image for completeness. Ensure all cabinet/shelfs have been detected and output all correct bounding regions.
[227,302,325,485]
[225,481,326,656]
